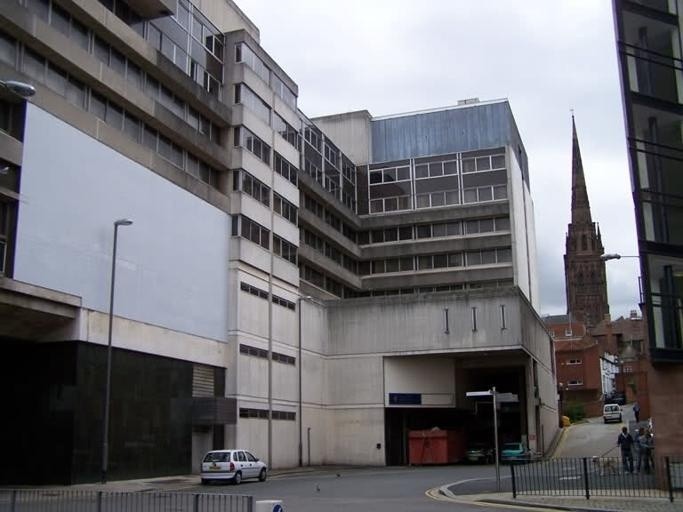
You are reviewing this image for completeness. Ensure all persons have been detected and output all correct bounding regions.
[617,427,655,475]
[633,400,640,423]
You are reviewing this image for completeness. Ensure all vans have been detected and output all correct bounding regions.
[602,403,623,423]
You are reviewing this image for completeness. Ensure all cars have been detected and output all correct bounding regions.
[200,449,268,485]
[464,441,531,465]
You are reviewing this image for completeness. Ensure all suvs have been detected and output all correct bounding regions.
[611,394,626,405]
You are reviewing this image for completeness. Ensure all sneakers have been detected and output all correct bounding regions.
[624,469,638,475]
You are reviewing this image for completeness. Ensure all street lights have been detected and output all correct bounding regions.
[101,218,133,484]
